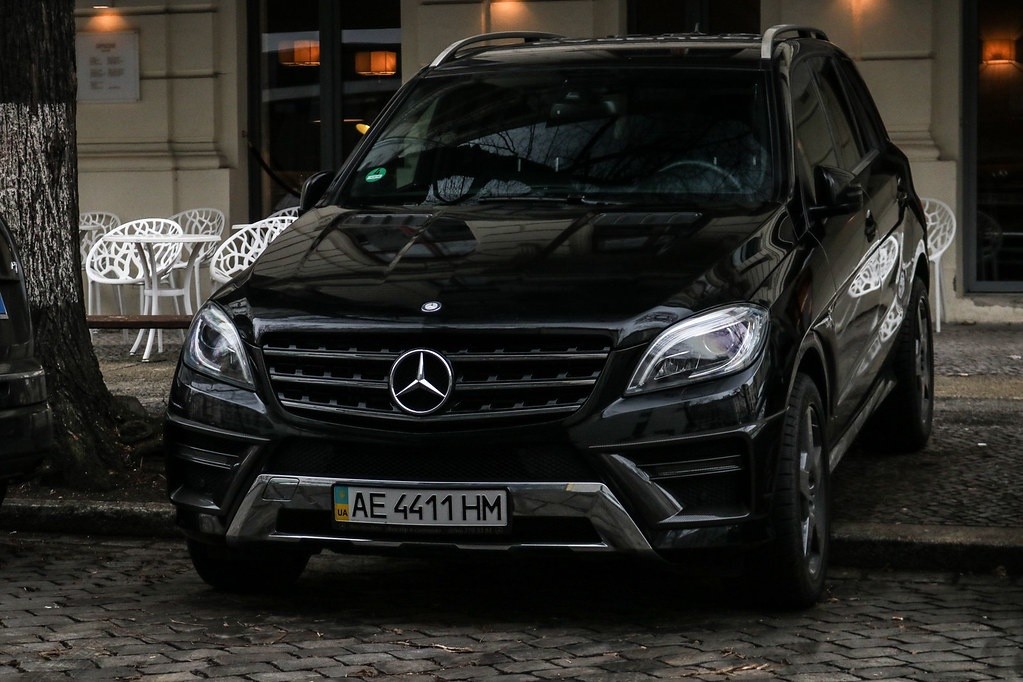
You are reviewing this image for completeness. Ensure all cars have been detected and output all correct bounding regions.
[0,209,57,506]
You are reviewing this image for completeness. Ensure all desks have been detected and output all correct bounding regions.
[101,230,221,364]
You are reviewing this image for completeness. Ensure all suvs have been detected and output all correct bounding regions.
[164,23,937,610]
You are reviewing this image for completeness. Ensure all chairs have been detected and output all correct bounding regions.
[75,207,301,343]
